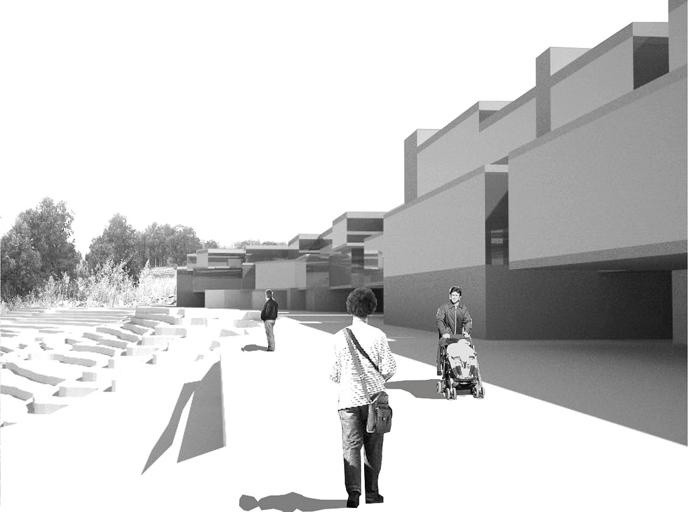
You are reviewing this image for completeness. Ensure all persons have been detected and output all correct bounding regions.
[260,289,279,351]
[329,287,396,508]
[435,287,476,380]
